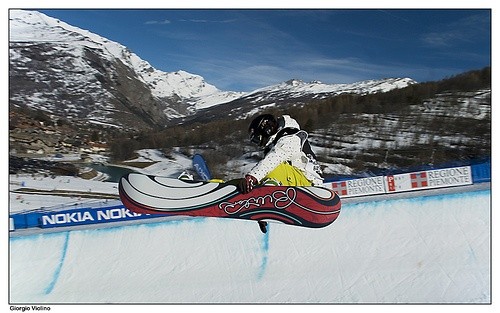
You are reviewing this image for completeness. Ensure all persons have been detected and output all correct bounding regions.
[238,113,324,233]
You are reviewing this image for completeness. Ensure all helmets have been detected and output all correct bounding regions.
[248,111,277,148]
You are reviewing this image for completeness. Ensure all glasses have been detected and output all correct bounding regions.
[250,118,268,145]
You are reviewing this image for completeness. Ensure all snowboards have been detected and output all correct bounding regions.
[118,173,341,229]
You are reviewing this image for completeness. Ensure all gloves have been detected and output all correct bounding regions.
[237,175,257,194]
